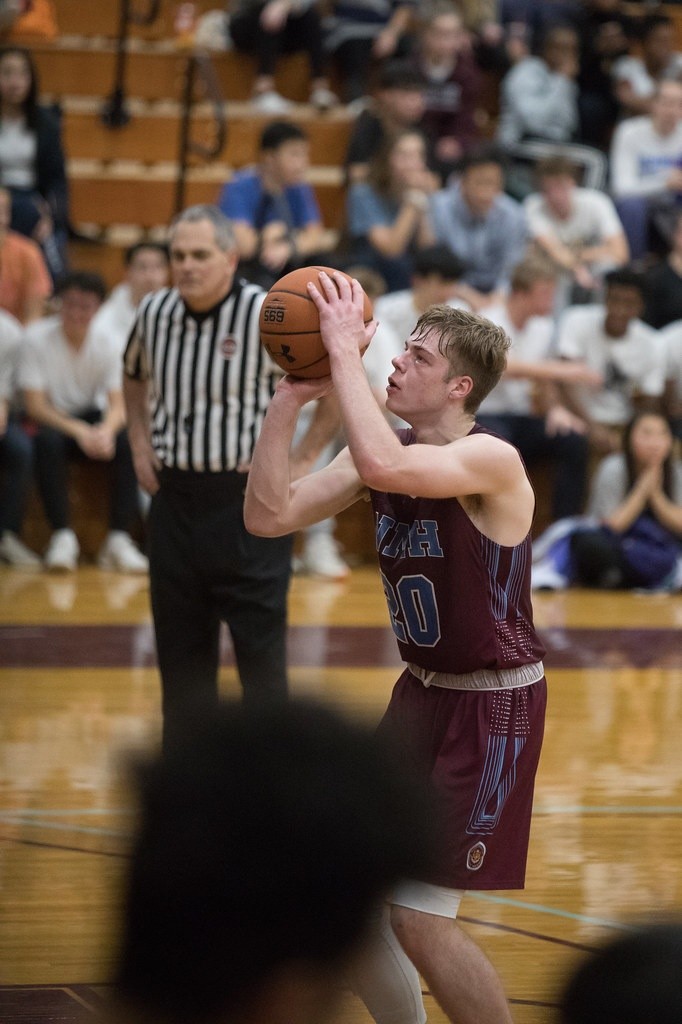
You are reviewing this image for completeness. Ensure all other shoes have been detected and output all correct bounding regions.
[0,533,41,568]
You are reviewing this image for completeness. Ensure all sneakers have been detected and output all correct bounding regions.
[98,531,149,574]
[46,529,80,571]
[302,533,351,580]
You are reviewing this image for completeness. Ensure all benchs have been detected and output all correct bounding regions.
[0,0,682,584]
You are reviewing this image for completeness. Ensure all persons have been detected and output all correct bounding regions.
[0,243,352,579]
[110,695,435,1024]
[123,203,344,751]
[362,239,682,593]
[0,0,682,329]
[243,270,548,1024]
[554,923,682,1024]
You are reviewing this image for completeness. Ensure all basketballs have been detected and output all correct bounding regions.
[258,265,374,379]
